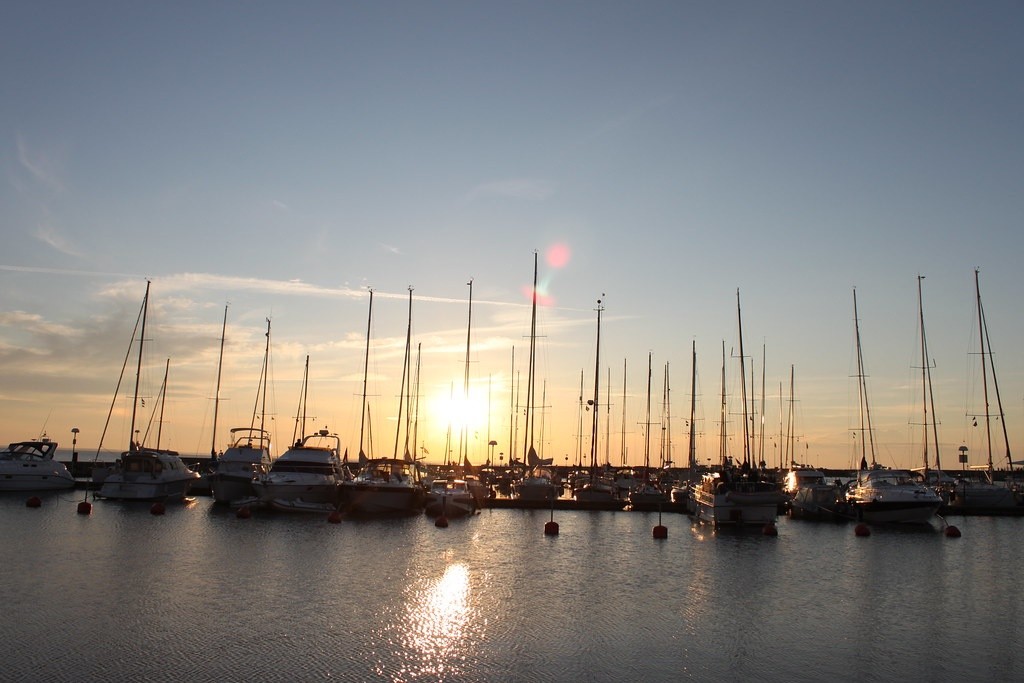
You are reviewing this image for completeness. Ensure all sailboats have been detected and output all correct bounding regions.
[98,250,1023,515]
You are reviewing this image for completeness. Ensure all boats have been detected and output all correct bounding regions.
[689,459,795,528]
[0,439,75,491]
[844,466,943,527]
